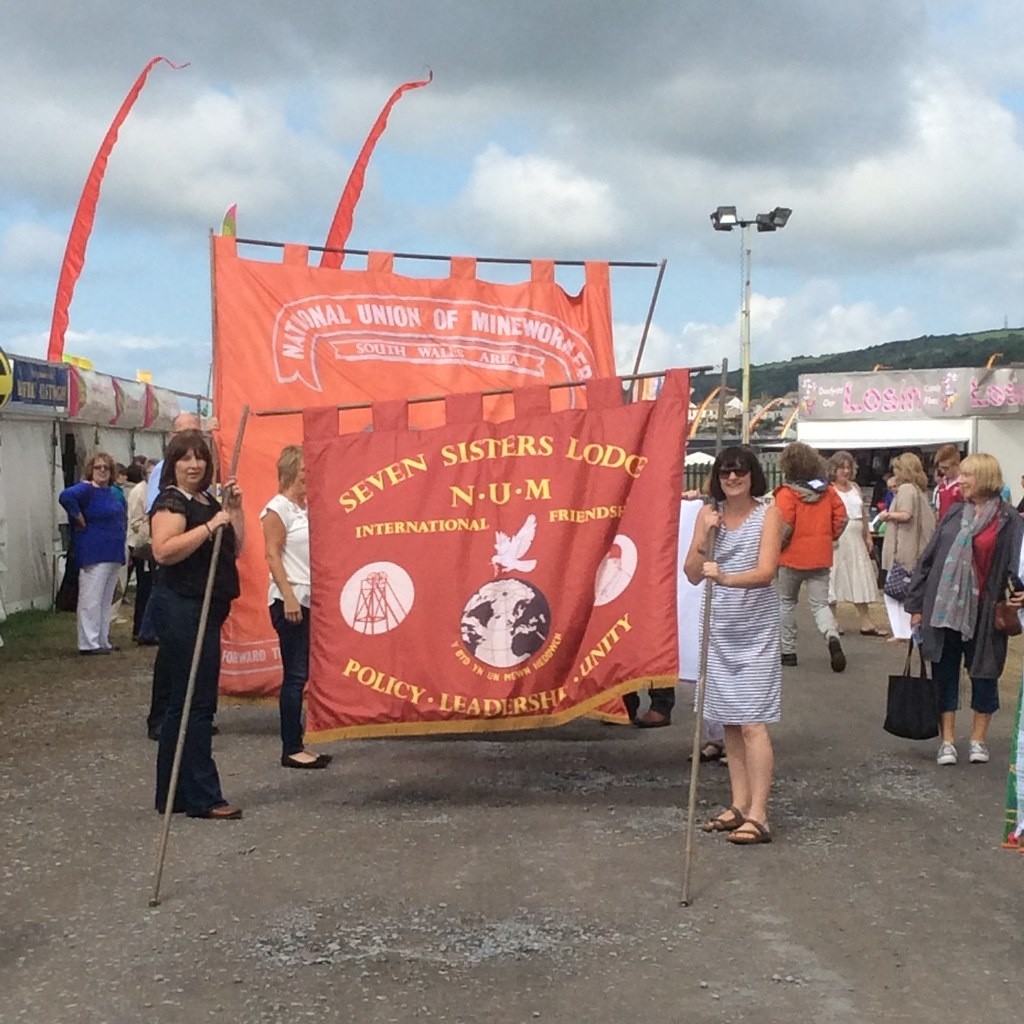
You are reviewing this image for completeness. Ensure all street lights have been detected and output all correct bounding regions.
[710,206,792,444]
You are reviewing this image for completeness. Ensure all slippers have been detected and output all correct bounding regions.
[702,807,744,831]
[728,820,773,843]
[861,626,887,637]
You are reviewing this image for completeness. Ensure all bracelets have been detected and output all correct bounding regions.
[204,523,213,542]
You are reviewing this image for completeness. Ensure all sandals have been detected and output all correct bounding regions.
[688,741,725,763]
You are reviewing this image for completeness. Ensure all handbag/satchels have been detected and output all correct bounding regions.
[882,630,940,740]
[884,560,915,602]
[994,601,1022,637]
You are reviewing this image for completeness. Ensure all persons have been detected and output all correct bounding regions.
[149,429,245,813]
[770,443,888,673]
[260,445,333,769]
[869,445,1024,766]
[58,453,160,655]
[145,412,220,743]
[598,447,782,844]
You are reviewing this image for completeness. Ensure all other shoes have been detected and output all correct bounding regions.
[829,637,846,673]
[781,654,797,665]
[79,648,110,655]
[201,803,241,818]
[635,710,669,727]
[211,725,219,734]
[282,753,332,768]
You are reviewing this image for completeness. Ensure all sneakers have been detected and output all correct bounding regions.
[968,739,989,763]
[937,741,957,764]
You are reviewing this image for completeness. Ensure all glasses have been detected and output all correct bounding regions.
[93,466,110,471]
[719,463,750,478]
